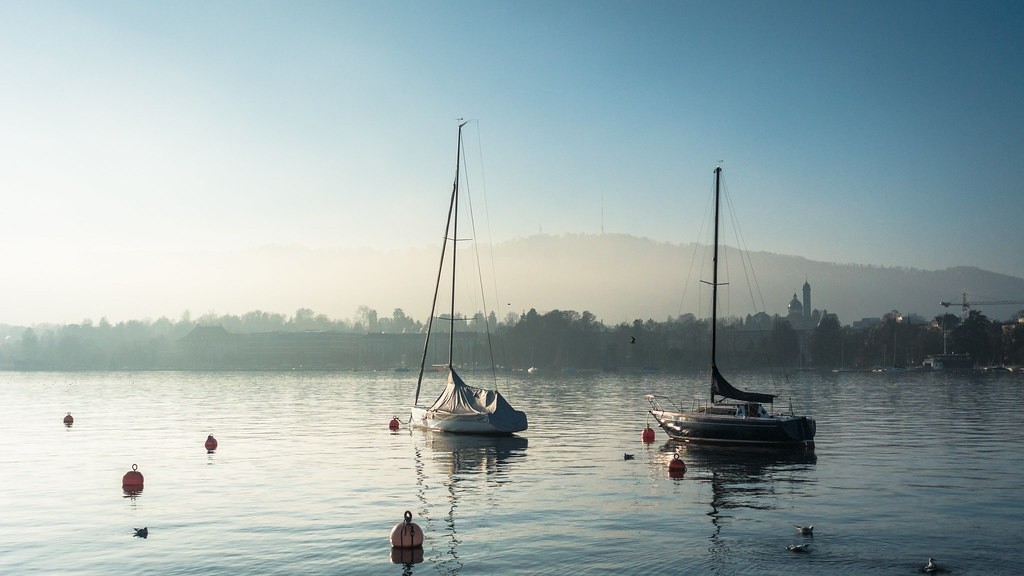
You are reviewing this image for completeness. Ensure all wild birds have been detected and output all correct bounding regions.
[922,557,937,570]
[133,526,147,534]
[630,336,636,344]
[623,452,635,459]
[787,544,812,552]
[794,524,815,533]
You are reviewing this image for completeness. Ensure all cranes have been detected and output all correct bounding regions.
[939,291,1024,321]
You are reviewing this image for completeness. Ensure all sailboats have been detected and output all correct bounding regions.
[831,311,1024,374]
[641,160,818,445]
[407,116,529,435]
